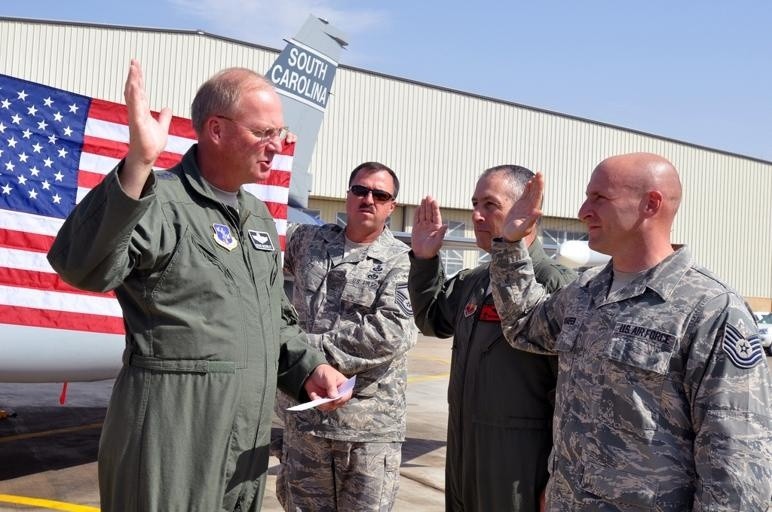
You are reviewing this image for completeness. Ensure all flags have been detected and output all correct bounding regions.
[0,71,299,342]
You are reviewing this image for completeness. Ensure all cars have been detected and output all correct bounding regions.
[753,312,772,355]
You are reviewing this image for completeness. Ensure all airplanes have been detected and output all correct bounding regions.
[1,10,351,407]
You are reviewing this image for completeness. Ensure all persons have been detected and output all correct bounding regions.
[487,149,770,510]
[48,51,355,512]
[279,130,424,512]
[403,160,582,512]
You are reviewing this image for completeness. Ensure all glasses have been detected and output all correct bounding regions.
[217,114,289,145]
[350,184,396,201]
